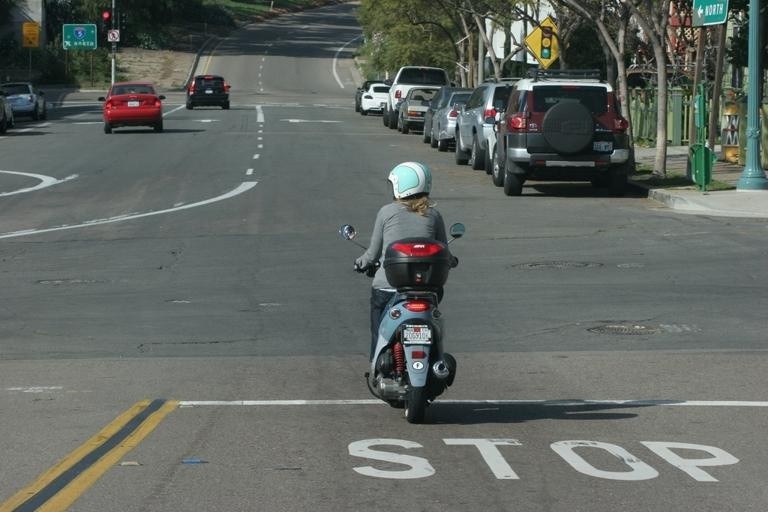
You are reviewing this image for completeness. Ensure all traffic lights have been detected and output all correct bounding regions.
[538,25,553,60]
[101,7,112,27]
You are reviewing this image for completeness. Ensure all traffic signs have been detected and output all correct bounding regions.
[689,0,728,26]
[62,23,97,50]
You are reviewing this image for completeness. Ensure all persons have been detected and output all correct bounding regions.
[354,162,458,363]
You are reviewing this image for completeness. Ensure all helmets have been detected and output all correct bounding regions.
[386,158,435,201]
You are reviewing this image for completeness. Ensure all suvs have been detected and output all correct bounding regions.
[183,73,230,111]
[378,65,457,129]
[488,69,634,200]
[0,82,49,120]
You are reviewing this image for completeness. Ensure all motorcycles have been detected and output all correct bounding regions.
[334,220,468,426]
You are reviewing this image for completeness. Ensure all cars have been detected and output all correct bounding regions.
[351,76,525,175]
[96,81,167,136]
[0,89,15,135]
